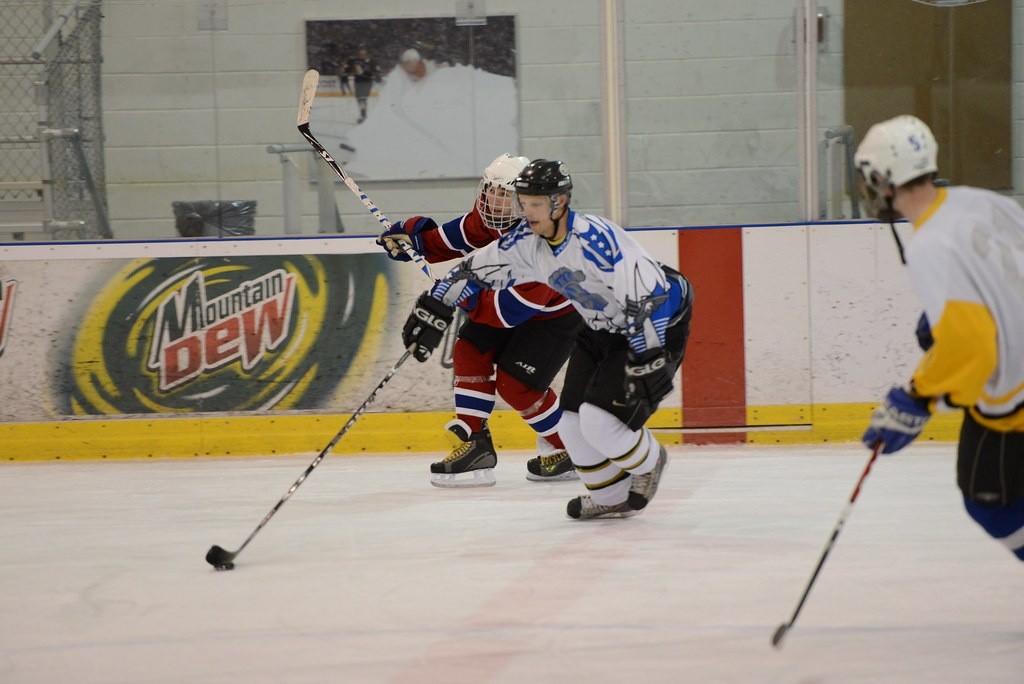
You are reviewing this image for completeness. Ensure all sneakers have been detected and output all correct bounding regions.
[431,419,497,473]
[567,495,645,518]
[628,446,667,510]
[527,451,575,481]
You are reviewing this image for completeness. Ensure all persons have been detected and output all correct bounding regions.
[855,114,1024,559]
[335,43,379,124]
[377,153,573,486]
[403,159,694,518]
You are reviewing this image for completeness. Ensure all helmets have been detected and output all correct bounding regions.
[515,159,573,196]
[475,152,529,192]
[853,115,938,220]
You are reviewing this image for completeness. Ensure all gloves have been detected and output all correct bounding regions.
[402,290,455,362]
[377,217,438,262]
[623,347,677,418]
[861,388,932,455]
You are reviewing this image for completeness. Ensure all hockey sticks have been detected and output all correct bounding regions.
[297,69,457,278]
[206,347,417,572]
[770,443,886,648]
